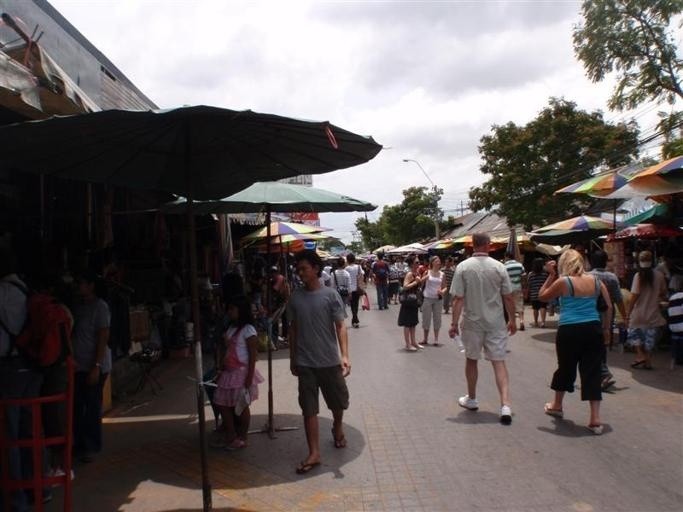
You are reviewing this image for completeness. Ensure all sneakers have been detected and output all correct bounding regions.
[405,345,416,351]
[458,395,480,410]
[544,402,563,418]
[415,344,423,349]
[499,405,512,424]
[585,422,604,434]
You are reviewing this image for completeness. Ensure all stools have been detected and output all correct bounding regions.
[126,344,166,400]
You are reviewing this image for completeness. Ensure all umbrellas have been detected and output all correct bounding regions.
[0,105,383,512]
[155,182,378,429]
[241,221,333,257]
[626,155,683,197]
[527,215,618,256]
[553,171,670,233]
[255,234,340,254]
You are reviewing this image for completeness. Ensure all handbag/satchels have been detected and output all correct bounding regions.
[399,290,417,304]
[417,288,424,307]
[337,288,348,297]
[356,265,362,295]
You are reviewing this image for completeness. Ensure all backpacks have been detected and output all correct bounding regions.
[0,272,71,369]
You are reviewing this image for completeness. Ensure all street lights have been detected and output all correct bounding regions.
[402,158,440,241]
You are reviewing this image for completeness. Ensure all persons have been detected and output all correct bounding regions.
[286,250,351,474]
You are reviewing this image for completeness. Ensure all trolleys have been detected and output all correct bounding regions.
[0,321,73,512]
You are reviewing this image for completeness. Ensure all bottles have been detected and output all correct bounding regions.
[613,325,621,344]
[454,333,465,354]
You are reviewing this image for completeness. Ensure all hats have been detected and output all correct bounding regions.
[637,251,652,268]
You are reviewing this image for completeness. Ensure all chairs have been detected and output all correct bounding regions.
[1,354,82,511]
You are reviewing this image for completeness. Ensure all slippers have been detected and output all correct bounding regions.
[600,375,615,392]
[331,428,346,448]
[630,359,646,367]
[296,461,320,475]
[225,438,247,450]
